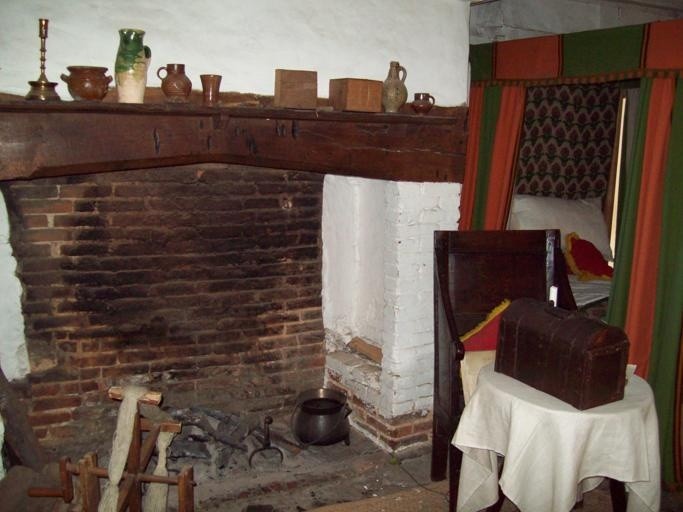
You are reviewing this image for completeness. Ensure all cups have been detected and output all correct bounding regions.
[200,74,222,106]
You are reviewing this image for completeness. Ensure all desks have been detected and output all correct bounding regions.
[450,362,662,512]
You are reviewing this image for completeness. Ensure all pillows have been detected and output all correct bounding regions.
[508,196,615,262]
[459,299,512,351]
[563,231,615,282]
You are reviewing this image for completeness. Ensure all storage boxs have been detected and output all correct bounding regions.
[494,297,630,411]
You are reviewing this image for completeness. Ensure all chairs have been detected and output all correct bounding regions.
[431,230,577,512]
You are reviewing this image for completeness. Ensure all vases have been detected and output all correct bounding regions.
[61,66,113,103]
[115,28,152,103]
[381,61,408,114]
